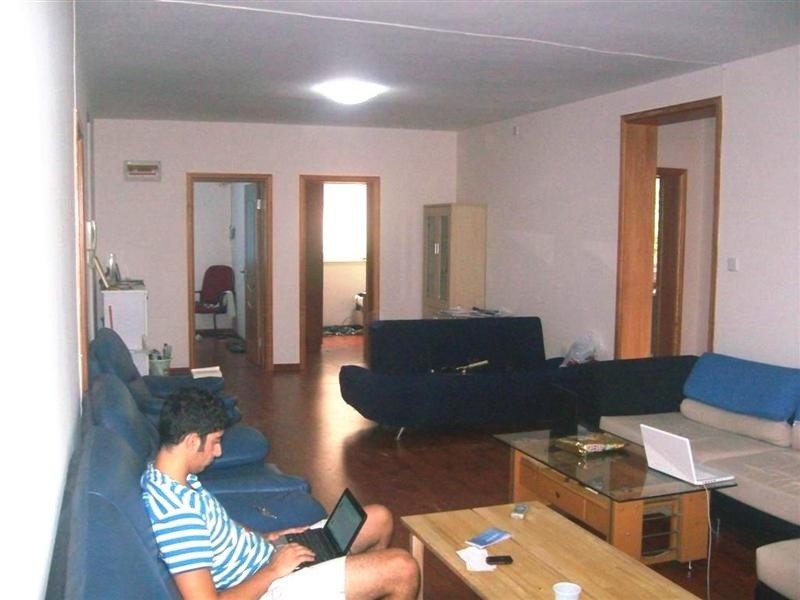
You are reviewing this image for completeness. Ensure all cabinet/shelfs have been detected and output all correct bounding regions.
[99,278,148,374]
[421,202,488,319]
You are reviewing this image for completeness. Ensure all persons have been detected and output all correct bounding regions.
[139,387,421,600]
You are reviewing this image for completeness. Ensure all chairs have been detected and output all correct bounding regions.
[191,265,238,339]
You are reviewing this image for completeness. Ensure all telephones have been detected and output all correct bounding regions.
[86,220,96,250]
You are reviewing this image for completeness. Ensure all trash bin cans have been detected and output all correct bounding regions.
[149,357,171,376]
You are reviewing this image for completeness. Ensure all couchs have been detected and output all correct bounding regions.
[599,352,800,600]
[335,314,567,443]
[38,323,330,599]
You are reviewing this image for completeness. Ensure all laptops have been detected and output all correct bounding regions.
[639,424,736,485]
[277,486,369,572]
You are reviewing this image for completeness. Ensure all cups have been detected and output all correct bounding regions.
[552,582,582,600]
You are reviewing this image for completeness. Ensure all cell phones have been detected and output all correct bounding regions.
[511,504,528,520]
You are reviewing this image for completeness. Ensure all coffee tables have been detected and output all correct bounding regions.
[490,420,737,571]
[397,499,701,600]
